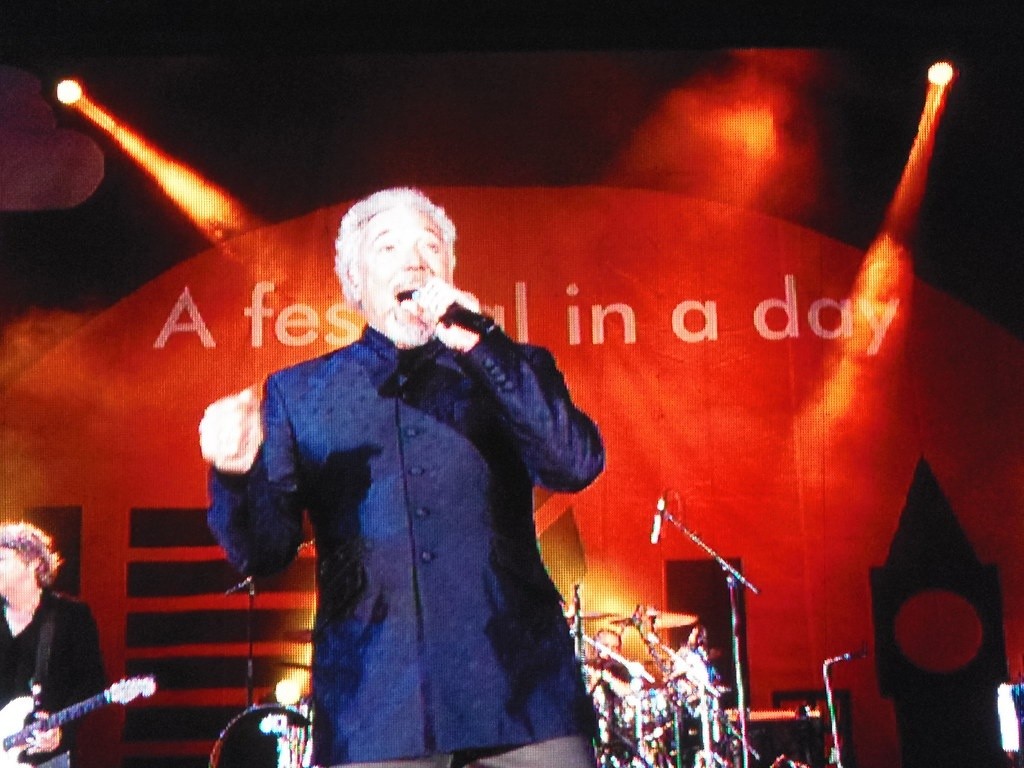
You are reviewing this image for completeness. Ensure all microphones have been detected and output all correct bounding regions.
[650,498,666,545]
[411,292,497,336]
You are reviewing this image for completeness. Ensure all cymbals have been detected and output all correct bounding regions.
[609,608,697,628]
[560,611,618,621]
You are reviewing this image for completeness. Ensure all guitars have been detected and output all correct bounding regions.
[1,673,158,768]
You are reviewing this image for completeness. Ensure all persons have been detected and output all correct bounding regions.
[0,523,107,768]
[197,184,606,768]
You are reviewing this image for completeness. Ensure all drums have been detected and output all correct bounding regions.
[208,703,313,768]
[590,673,824,768]
